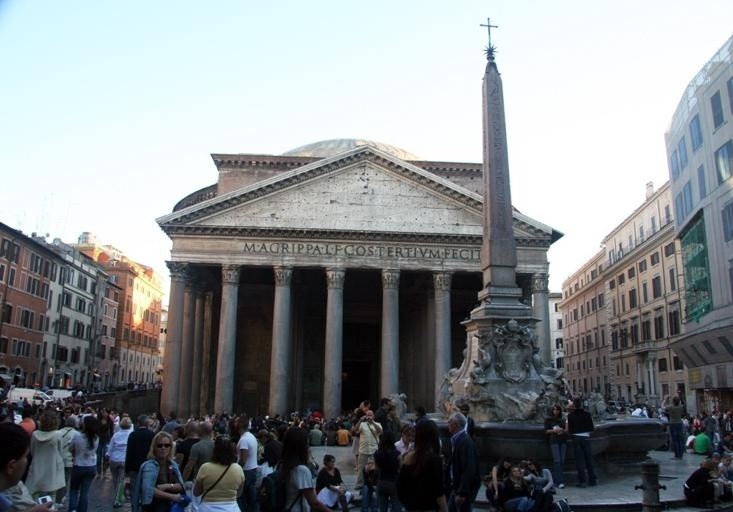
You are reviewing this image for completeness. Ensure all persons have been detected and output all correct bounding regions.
[1,377,732,512]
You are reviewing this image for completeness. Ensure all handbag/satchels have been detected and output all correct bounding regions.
[170,492,192,512]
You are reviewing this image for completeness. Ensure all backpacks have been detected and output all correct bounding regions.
[258,460,303,512]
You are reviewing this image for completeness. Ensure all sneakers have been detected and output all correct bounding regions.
[558,483,564,489]
[588,479,599,485]
[576,482,585,487]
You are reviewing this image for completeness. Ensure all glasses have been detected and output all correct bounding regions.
[157,443,171,448]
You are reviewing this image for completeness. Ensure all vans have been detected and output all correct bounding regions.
[45,389,75,403]
[4,387,54,407]
